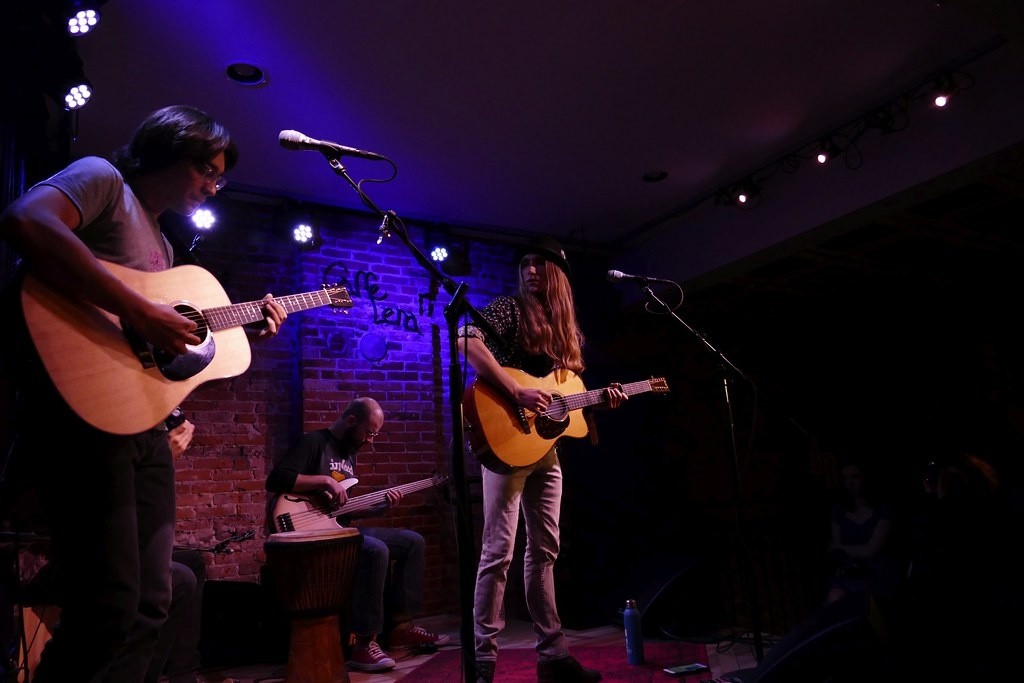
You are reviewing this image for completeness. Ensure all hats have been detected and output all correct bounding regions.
[512,234,570,276]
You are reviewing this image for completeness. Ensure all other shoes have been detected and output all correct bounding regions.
[476,660,494,683]
[537,656,601,683]
[169,670,210,683]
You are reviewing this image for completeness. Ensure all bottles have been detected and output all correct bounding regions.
[623,599,644,666]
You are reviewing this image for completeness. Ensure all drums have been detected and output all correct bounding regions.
[263,532,366,683]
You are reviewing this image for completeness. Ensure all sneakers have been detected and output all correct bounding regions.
[350,640,395,671]
[389,622,449,651]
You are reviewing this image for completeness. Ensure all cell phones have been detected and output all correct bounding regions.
[663,663,710,676]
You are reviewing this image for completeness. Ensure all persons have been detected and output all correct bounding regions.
[8,344,210,683]
[264,397,449,672]
[455,233,629,683]
[825,456,894,603]
[0,101,288,683]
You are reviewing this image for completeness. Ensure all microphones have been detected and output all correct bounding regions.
[241,530,256,541]
[607,270,677,286]
[278,129,385,160]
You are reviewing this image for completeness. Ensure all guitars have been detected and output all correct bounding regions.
[462,366,672,478]
[13,264,357,442]
[267,470,450,534]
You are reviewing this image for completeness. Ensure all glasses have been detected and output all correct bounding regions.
[198,159,227,191]
[357,421,379,439]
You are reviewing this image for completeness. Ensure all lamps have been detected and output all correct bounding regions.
[37,0,101,111]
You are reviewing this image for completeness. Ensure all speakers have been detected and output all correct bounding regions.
[616,556,725,640]
[745,596,881,683]
